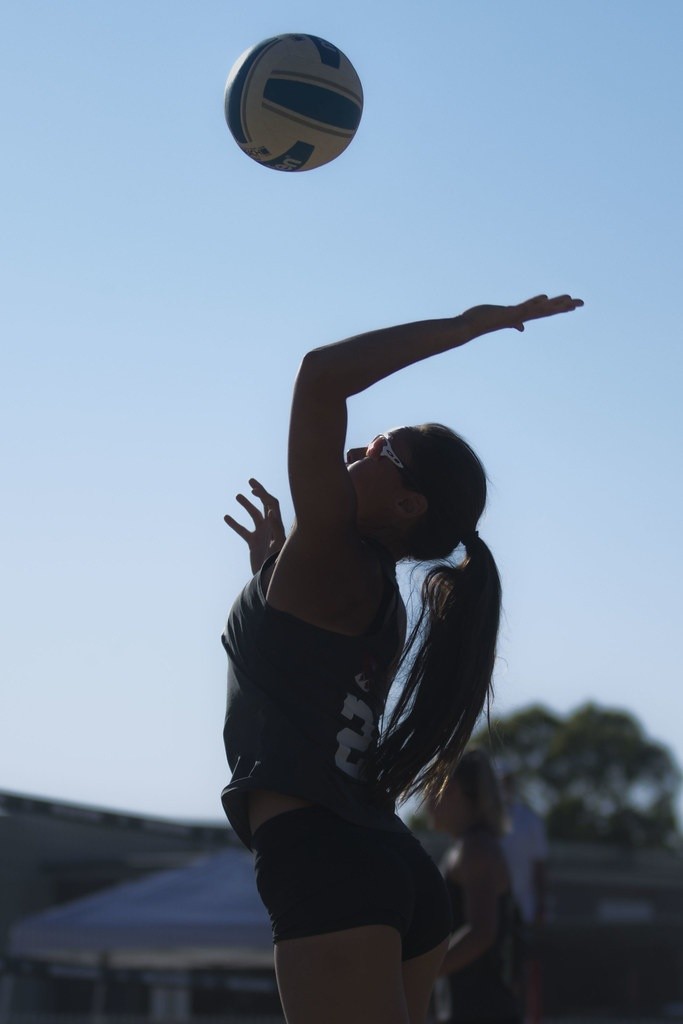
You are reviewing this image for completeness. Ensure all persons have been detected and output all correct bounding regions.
[217,292,589,1023]
[422,742,522,1024]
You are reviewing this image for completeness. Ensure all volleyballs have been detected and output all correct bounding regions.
[222,32,366,173]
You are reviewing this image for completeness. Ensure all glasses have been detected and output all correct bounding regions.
[365,433,424,497]
